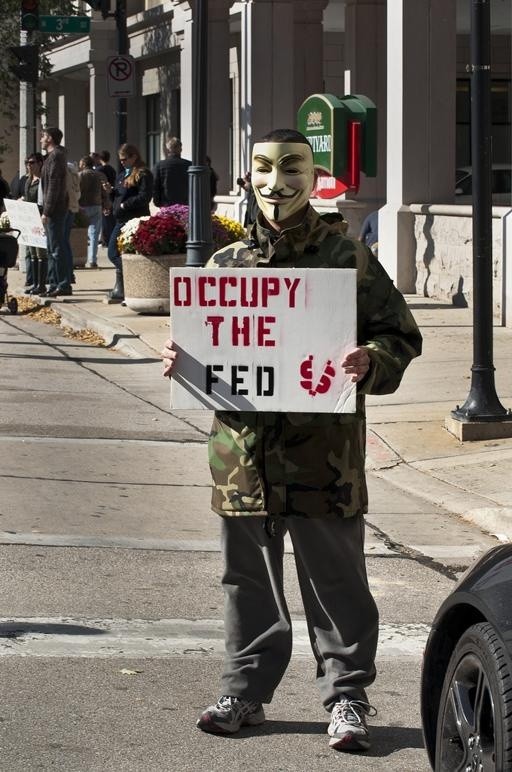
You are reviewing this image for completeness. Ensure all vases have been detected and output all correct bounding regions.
[68,228,88,267]
[121,251,186,313]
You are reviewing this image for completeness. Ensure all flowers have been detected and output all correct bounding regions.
[71,206,91,228]
[117,203,248,254]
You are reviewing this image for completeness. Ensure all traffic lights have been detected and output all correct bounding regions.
[21,0,40,31]
[4,43,40,84]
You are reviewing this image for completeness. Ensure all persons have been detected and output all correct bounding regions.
[152,137,192,208]
[90,153,101,167]
[157,127,426,754]
[77,155,111,268]
[2,152,48,295]
[40,127,73,296]
[102,143,154,298]
[207,156,217,211]
[66,161,80,284]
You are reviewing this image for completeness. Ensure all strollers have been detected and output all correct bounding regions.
[0,228,21,315]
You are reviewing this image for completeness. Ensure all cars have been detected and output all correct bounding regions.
[420,542,512,772]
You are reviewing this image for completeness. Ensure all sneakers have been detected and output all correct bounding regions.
[327,702,371,754]
[21,282,124,299]
[196,691,269,734]
